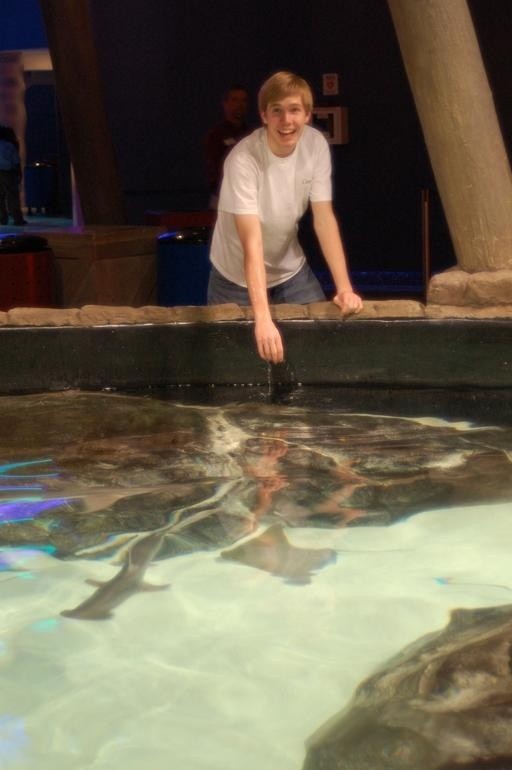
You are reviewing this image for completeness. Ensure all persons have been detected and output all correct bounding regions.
[206,70,364,365]
[203,90,250,210]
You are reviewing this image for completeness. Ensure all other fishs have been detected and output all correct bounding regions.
[0,389,512,622]
